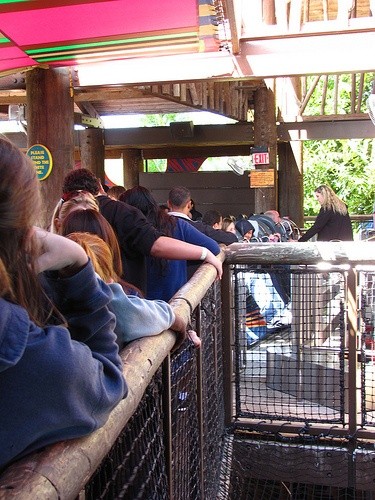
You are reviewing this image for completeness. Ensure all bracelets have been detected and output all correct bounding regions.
[200,247,206,260]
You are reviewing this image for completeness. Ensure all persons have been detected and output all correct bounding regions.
[287,185,354,242]
[0,133,128,473]
[47,167,300,411]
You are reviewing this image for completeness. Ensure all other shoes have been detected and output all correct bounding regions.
[279,310,292,324]
[267,316,292,328]
